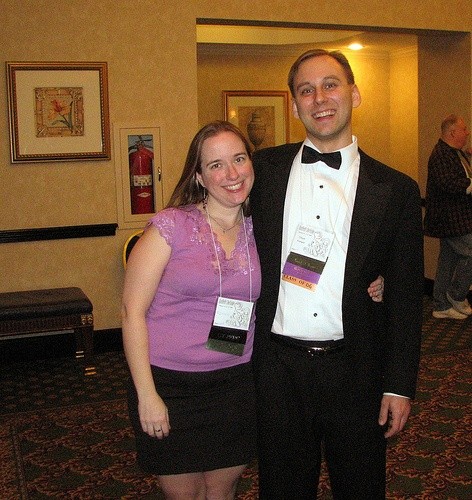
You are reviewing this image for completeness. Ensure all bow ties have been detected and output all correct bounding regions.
[301,145,341,170]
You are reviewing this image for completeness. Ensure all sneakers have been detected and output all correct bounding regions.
[433,308,468,319]
[447,291,472,315]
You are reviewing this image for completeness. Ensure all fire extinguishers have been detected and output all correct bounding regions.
[129,136,154,214]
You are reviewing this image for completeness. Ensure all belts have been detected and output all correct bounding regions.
[271,333,345,357]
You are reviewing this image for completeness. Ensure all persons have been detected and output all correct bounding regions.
[423,114,472,319]
[245,49,422,500]
[121,120,386,499]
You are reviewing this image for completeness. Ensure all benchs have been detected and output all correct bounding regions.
[0,287,97,377]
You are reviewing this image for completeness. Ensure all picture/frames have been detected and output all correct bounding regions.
[5,58,111,164]
[221,89,289,149]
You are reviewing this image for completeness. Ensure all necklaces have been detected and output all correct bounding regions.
[207,213,242,233]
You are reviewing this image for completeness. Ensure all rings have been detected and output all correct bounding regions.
[155,429,162,433]
